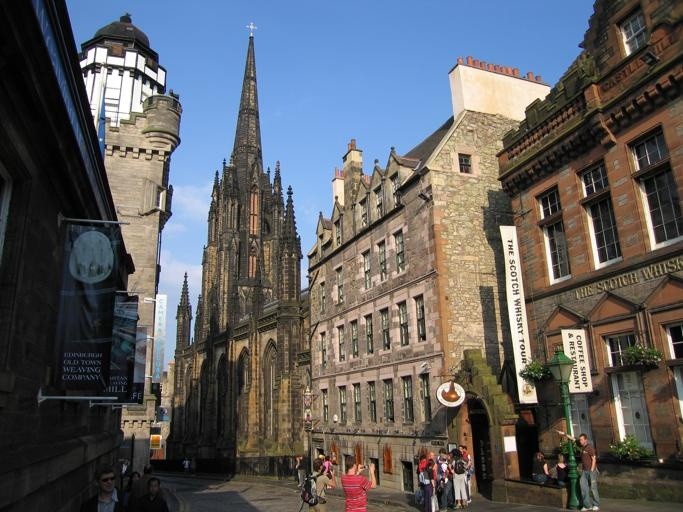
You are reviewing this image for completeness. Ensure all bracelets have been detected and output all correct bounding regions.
[564,432,567,436]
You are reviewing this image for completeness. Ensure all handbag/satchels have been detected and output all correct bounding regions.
[430,489,438,512]
[414,487,424,505]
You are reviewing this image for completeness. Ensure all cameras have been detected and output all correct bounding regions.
[361,465,369,470]
[327,465,334,470]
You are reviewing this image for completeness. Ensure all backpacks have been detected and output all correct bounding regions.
[451,457,463,475]
[418,469,432,487]
[298,473,326,512]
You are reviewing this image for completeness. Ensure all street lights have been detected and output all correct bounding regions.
[546,345,583,511]
[302,386,316,482]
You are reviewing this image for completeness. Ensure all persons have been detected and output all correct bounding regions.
[341,456,377,512]
[556,430,601,511]
[182,457,190,474]
[532,451,558,485]
[416,445,475,512]
[556,453,570,486]
[295,454,337,512]
[80,465,170,512]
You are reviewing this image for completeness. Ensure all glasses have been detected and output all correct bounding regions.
[101,477,114,482]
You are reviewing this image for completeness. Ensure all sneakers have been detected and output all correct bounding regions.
[462,503,466,509]
[592,506,598,511]
[453,504,462,510]
[580,507,591,512]
